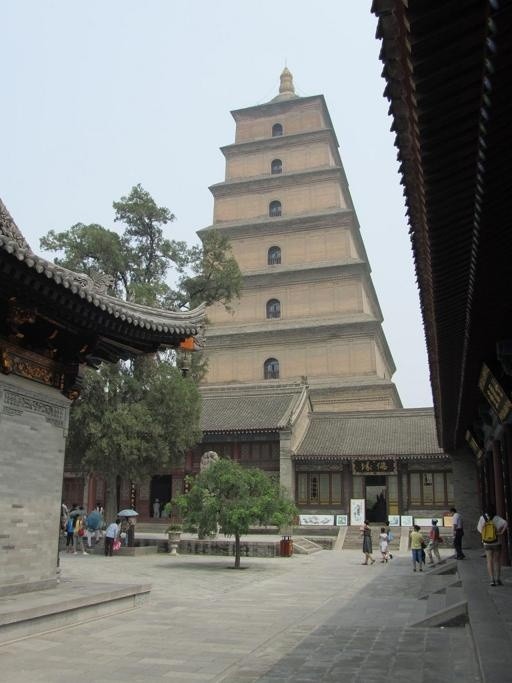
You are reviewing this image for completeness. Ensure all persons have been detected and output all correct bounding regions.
[377,527,389,563]
[358,519,376,565]
[426,517,441,564]
[384,521,392,560]
[476,505,508,585]
[407,525,425,571]
[60,500,137,556]
[479,509,486,557]
[449,507,465,560]
[152,498,161,517]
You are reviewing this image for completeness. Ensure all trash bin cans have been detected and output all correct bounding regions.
[279,536,293,557]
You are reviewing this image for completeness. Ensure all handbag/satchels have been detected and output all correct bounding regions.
[78,528,85,536]
[438,538,442,543]
[422,544,427,549]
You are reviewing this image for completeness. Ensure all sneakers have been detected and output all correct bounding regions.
[489,580,502,586]
[362,558,388,565]
[456,555,465,560]
[74,551,89,555]
[413,568,423,572]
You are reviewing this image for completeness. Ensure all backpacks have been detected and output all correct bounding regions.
[482,519,497,544]
